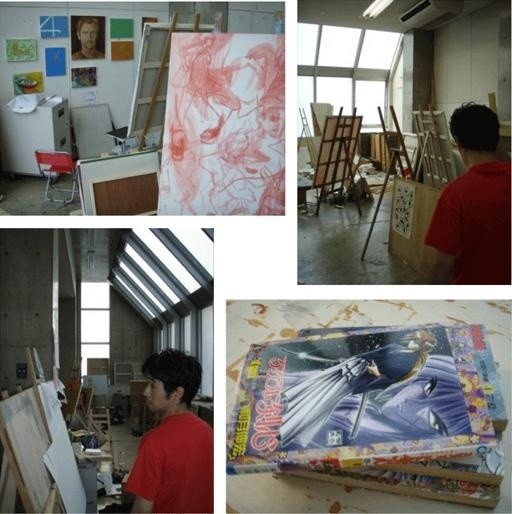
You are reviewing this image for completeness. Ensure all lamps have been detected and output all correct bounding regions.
[360,0,394,20]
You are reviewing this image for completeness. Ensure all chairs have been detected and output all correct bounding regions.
[34,150,77,205]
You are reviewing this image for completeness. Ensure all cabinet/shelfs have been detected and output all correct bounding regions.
[2,97,74,186]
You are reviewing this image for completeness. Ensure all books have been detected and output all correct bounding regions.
[225,319,509,511]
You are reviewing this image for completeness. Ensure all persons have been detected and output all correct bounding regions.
[71,18,105,61]
[124,347,215,513]
[421,100,511,285]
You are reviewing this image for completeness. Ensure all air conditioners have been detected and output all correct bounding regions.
[398,0,461,30]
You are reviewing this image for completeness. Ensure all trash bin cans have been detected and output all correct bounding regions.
[130,407,145,437]
